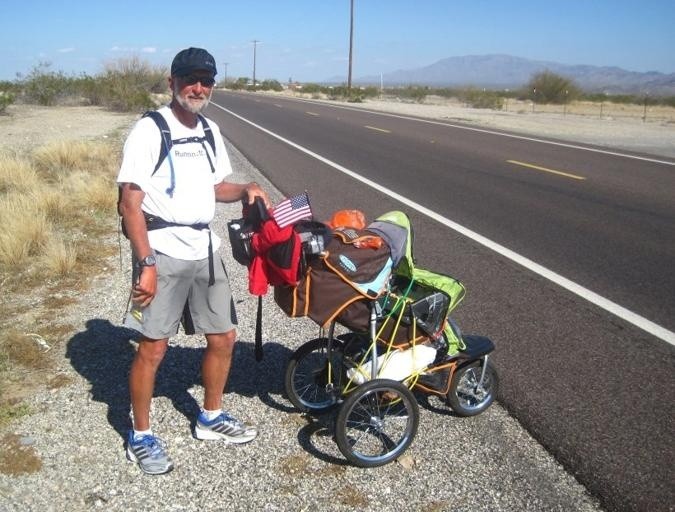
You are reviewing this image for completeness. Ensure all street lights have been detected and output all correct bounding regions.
[251,39,260,87]
[223,63,229,83]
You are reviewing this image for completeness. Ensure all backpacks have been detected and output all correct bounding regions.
[118,111,216,240]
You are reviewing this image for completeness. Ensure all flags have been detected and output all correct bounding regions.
[272,192,313,229]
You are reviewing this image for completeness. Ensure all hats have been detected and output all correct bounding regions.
[171,47,218,75]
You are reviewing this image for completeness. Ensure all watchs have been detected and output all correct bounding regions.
[137,255,156,268]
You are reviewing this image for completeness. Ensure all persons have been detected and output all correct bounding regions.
[116,48,275,474]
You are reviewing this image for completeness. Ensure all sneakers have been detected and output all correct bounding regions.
[127,430,174,475]
[193,412,258,444]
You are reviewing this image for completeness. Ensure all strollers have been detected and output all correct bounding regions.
[241,192,500,467]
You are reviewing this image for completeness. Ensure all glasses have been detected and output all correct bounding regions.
[178,76,215,87]
